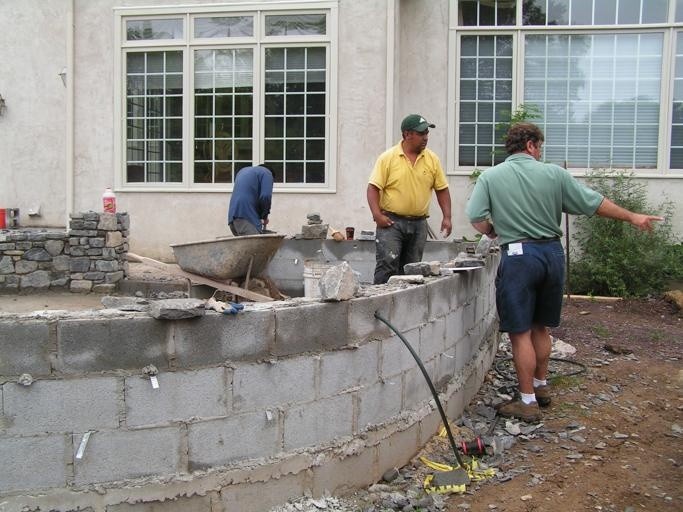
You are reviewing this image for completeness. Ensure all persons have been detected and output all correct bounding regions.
[227,163,278,238]
[463,124,663,424]
[202,121,240,183]
[366,113,452,286]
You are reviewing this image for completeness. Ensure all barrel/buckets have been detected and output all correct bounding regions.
[102,188,117,214]
[303,263,333,298]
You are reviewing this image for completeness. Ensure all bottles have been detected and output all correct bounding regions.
[103,186,116,215]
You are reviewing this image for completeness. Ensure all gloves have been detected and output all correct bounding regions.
[204,297,243,314]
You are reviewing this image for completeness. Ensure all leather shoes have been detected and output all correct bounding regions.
[493,394,542,423]
[513,384,551,406]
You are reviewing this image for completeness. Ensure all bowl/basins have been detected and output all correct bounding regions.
[171,235,285,281]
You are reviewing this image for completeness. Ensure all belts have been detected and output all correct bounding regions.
[381,209,429,221]
[515,236,559,243]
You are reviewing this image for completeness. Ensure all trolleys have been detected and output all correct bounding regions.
[125,233,291,302]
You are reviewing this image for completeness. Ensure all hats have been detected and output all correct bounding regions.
[401,114,435,132]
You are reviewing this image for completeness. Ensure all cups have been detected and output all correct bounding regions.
[345,227,356,240]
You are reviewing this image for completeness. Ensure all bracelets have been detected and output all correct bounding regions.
[485,224,493,235]
